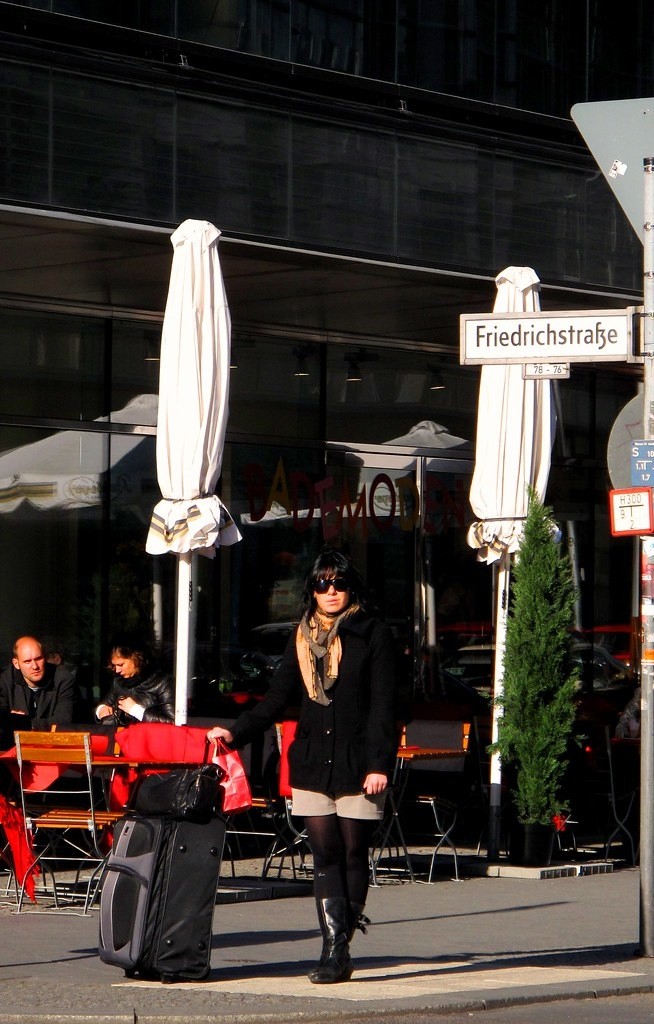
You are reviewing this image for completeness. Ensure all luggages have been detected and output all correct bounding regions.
[95,737,226,982]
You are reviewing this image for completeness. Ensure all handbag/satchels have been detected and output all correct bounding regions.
[211,737,252,814]
[136,759,226,824]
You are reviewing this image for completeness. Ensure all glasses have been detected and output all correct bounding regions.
[313,579,349,593]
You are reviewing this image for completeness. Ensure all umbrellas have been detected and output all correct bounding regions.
[464,266,565,865]
[143,217,245,725]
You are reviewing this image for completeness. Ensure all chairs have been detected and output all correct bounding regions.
[0,720,472,914]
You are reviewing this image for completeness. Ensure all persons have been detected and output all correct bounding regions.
[227,549,409,985]
[0,633,93,869]
[95,631,177,858]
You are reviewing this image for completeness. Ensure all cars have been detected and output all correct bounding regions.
[246,622,299,670]
[434,643,636,704]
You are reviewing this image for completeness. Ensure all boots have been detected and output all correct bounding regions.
[308,898,372,984]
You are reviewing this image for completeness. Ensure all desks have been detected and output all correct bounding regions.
[4,747,137,901]
